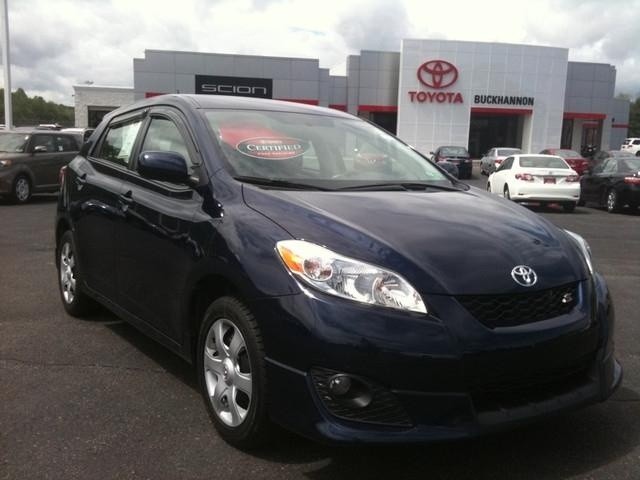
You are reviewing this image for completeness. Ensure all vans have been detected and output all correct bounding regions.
[0,131,84,202]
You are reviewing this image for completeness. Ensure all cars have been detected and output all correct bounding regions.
[588,150,634,173]
[353,142,394,172]
[540,148,588,176]
[575,156,640,213]
[486,153,582,213]
[56,94,623,444]
[0,123,95,143]
[429,144,473,179]
[479,147,526,175]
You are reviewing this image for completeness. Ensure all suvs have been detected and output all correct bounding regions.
[621,137,640,158]
